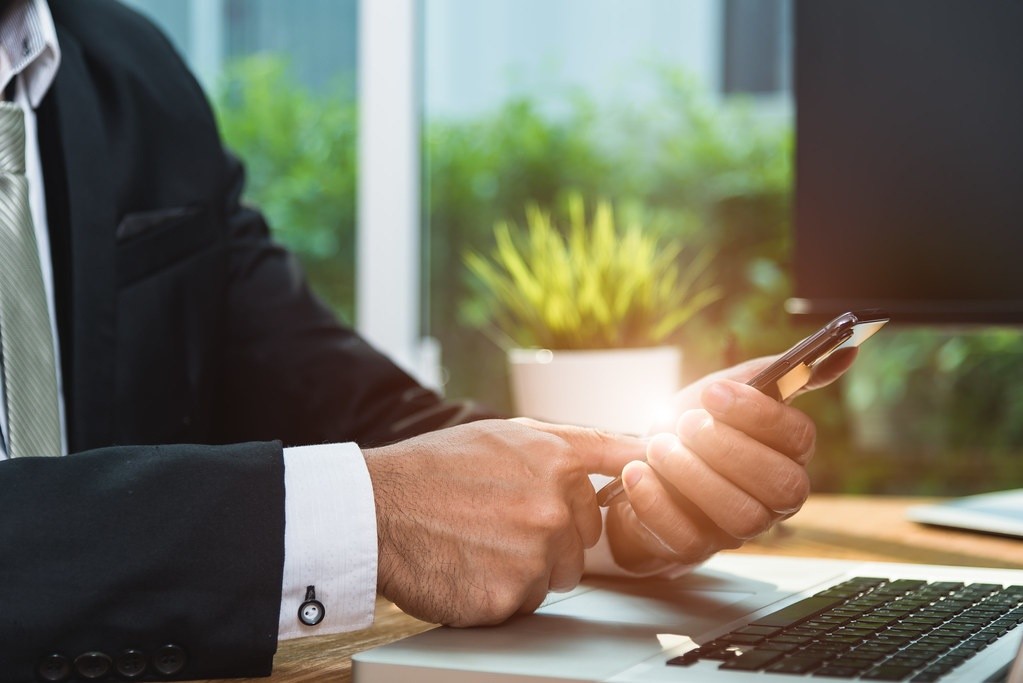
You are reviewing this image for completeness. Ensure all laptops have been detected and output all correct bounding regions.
[351,553,1023,683]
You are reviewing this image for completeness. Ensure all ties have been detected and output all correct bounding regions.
[0,100,71,458]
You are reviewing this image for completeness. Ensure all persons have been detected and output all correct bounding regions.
[0,0,817,683]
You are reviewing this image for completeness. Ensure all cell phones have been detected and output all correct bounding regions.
[593,309,890,508]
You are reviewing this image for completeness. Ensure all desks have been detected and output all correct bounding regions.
[268,495,1022,683]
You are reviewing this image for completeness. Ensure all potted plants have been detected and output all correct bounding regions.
[457,195,718,568]
